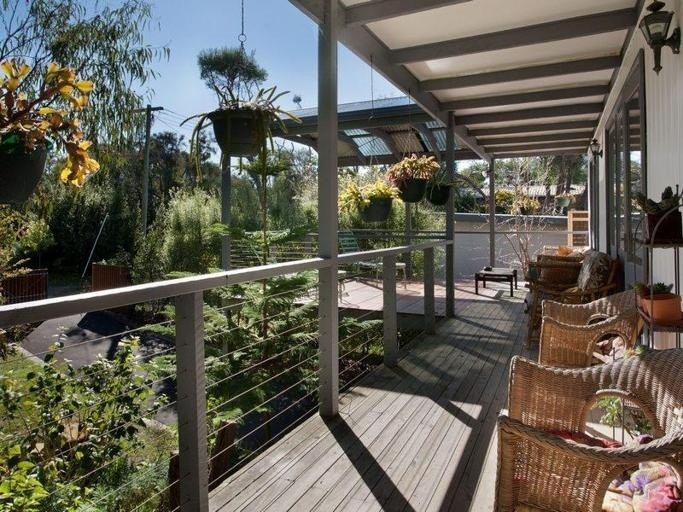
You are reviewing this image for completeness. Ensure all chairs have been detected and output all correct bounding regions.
[523,244,618,348]
[538,287,635,368]
[494,347,683,512]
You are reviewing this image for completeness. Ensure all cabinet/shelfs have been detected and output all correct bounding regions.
[633,240,683,350]
[568,211,589,247]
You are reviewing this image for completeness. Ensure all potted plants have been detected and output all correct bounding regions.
[631,183,683,244]
[630,280,667,308]
[0,55,101,200]
[555,192,577,207]
[426,159,474,205]
[338,174,403,227]
[178,48,303,187]
[383,153,441,203]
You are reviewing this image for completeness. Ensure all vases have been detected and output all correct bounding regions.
[644,294,682,326]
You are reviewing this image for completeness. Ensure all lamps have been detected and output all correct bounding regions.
[637,0,680,75]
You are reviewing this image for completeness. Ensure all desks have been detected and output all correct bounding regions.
[475,268,518,297]
[356,261,407,290]
